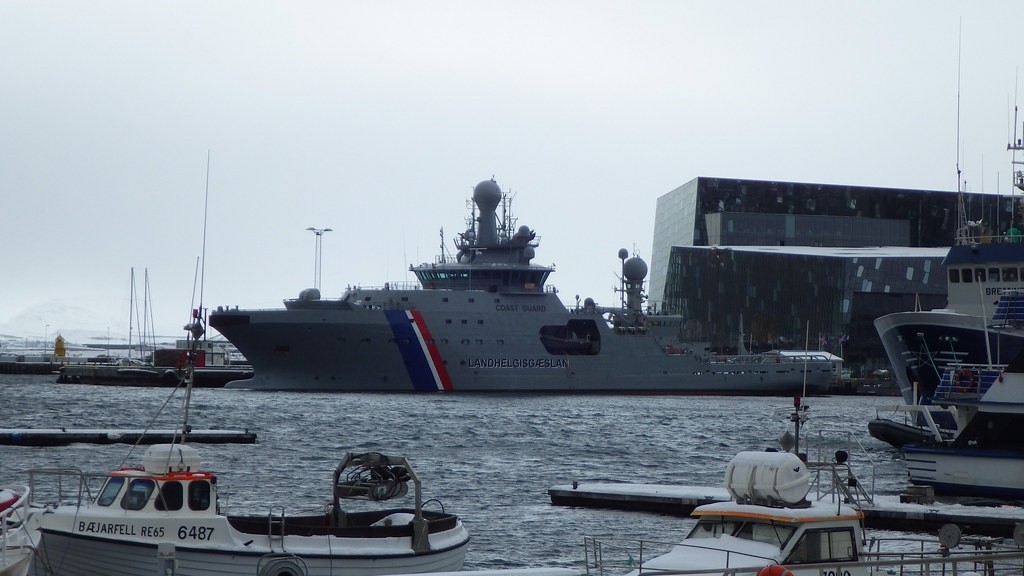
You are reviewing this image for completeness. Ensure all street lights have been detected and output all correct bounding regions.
[45,324,50,356]
[108,327,110,356]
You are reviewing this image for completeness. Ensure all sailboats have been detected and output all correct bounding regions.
[869,276,1008,449]
[20,146,472,575]
[0,257,254,387]
[872,13,1023,425]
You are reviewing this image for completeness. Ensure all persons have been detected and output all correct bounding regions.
[980,223,1021,243]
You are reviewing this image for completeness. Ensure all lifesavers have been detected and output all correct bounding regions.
[955,369,974,392]
[754,565,794,576]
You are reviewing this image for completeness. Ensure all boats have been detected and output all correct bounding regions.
[582,319,1024,575]
[0,480,41,576]
[209,176,834,393]
[901,344,1024,502]
